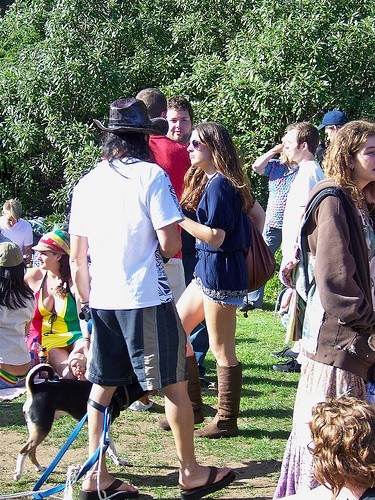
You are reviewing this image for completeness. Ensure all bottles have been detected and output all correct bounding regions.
[30,339,41,365]
[38,347,49,379]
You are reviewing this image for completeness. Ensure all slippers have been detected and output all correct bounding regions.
[79,478,139,500]
[179,466,236,499]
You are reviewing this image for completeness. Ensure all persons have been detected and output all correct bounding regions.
[307,396,375,499]
[21,228,89,380]
[126,89,206,411]
[238,136,299,311]
[271,124,327,374]
[160,123,266,438]
[317,108,347,144]
[1,240,34,392]
[276,121,375,499]
[1,198,34,270]
[68,96,236,499]
[163,96,212,392]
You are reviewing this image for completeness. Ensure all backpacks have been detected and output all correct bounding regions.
[275,188,352,345]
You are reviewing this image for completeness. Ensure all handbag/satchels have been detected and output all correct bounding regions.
[244,212,277,294]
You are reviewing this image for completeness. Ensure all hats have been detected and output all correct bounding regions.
[92,99,169,136]
[317,111,347,131]
[31,230,71,257]
[0,242,24,267]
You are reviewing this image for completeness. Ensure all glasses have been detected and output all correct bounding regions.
[186,139,210,151]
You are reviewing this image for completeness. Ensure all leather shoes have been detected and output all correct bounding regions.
[270,346,302,373]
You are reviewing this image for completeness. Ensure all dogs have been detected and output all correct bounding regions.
[13,363,158,482]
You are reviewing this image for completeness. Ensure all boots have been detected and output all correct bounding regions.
[159,350,242,437]
